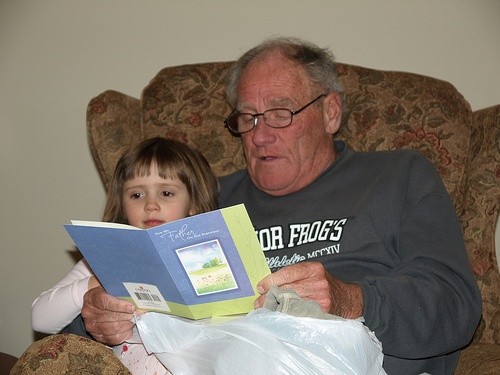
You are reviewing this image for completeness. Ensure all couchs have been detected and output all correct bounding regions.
[7,61,500,375]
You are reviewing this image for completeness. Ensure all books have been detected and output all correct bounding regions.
[62,202,273,320]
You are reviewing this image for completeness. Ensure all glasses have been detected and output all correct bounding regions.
[224,94,327,134]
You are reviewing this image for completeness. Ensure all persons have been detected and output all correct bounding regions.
[61,36,483,375]
[31,136,219,339]
